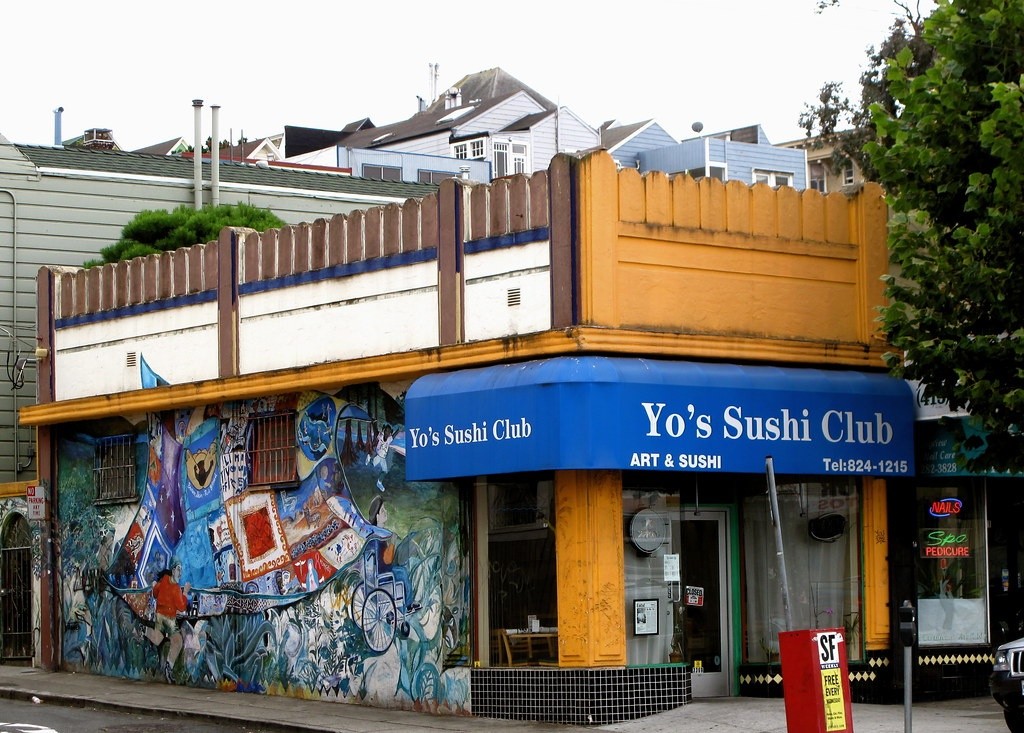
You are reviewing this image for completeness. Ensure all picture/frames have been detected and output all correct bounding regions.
[633,598,660,635]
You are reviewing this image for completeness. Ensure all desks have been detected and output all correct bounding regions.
[510,632,557,666]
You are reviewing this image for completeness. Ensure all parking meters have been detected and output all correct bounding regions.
[898,599,917,733]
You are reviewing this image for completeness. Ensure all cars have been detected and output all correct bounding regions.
[988,637,1024,733]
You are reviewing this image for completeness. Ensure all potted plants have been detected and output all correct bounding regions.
[760,633,780,662]
[669,637,684,663]
[845,614,862,659]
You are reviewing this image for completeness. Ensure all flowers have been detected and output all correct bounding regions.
[809,582,834,629]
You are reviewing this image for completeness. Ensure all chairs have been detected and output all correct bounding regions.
[490,629,559,667]
